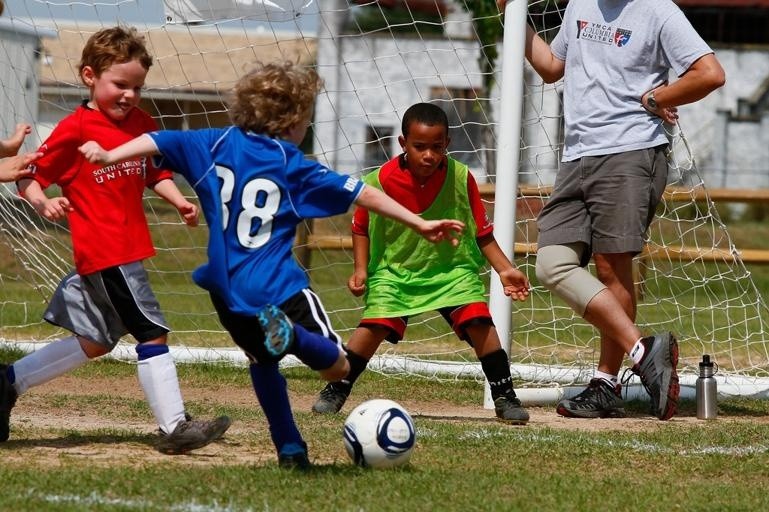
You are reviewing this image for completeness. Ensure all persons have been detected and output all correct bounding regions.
[76,59,468,470]
[491,0,726,421]
[0,117,47,184]
[309,101,530,426]
[0,25,231,454]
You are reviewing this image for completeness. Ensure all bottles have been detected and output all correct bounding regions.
[695,354,720,418]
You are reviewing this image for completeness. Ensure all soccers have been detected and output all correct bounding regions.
[344,397,415,469]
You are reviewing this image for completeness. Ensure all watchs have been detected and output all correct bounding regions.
[647,91,659,112]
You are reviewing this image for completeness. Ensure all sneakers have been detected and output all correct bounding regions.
[278,445,311,472]
[0,364,18,444]
[495,397,530,424]
[555,378,626,418]
[159,413,230,455]
[621,331,680,421]
[258,303,294,357]
[312,379,350,414]
